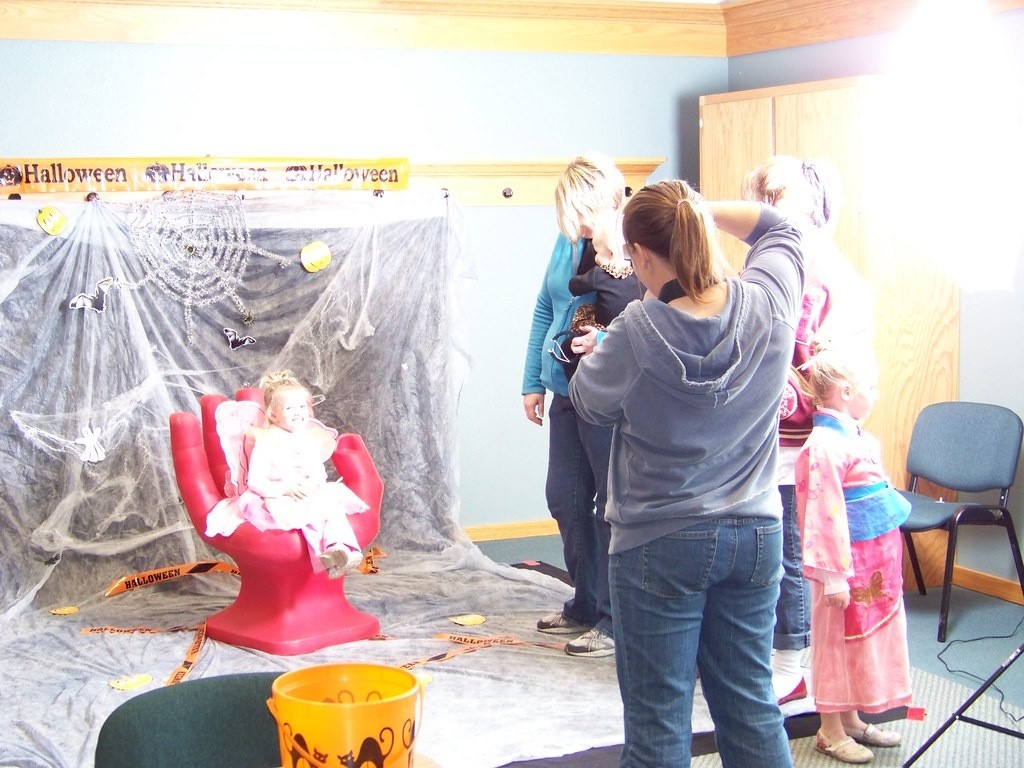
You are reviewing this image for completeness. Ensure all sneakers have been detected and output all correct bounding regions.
[536,611,593,632]
[564,629,617,657]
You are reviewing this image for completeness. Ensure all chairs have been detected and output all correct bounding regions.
[893,402,1024,642]
[171,390,385,655]
[95,673,287,767]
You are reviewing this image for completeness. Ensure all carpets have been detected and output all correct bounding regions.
[691,647,1024,768]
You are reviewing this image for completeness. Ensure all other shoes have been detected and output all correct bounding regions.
[816,729,874,762]
[328,552,363,579]
[845,721,900,745]
[319,544,351,568]
[777,677,807,705]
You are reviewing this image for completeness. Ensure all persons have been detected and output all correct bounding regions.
[794,341,914,764]
[521,159,648,659]
[567,177,808,768]
[205,368,370,579]
[742,154,879,707]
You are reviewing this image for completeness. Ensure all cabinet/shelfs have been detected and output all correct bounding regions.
[700,75,961,591]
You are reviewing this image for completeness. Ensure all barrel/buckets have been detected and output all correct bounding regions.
[266,663,425,768]
